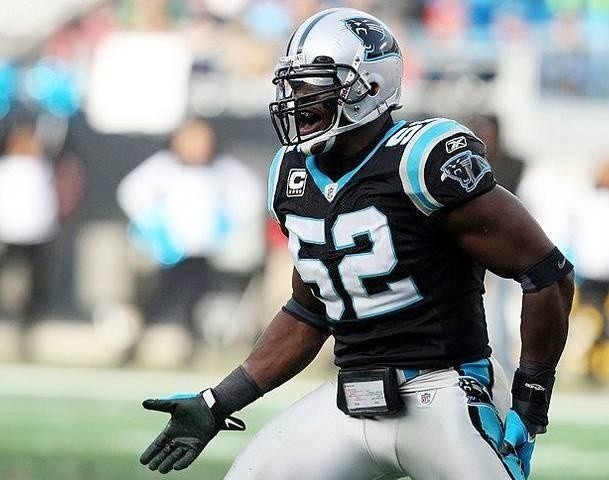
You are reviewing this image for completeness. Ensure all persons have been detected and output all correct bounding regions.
[1,0,608,394]
[143,7,573,480]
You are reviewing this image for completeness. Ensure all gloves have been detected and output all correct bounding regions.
[140,390,227,472]
[501,369,555,479]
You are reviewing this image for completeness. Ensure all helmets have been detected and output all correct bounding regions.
[270,7,404,156]
